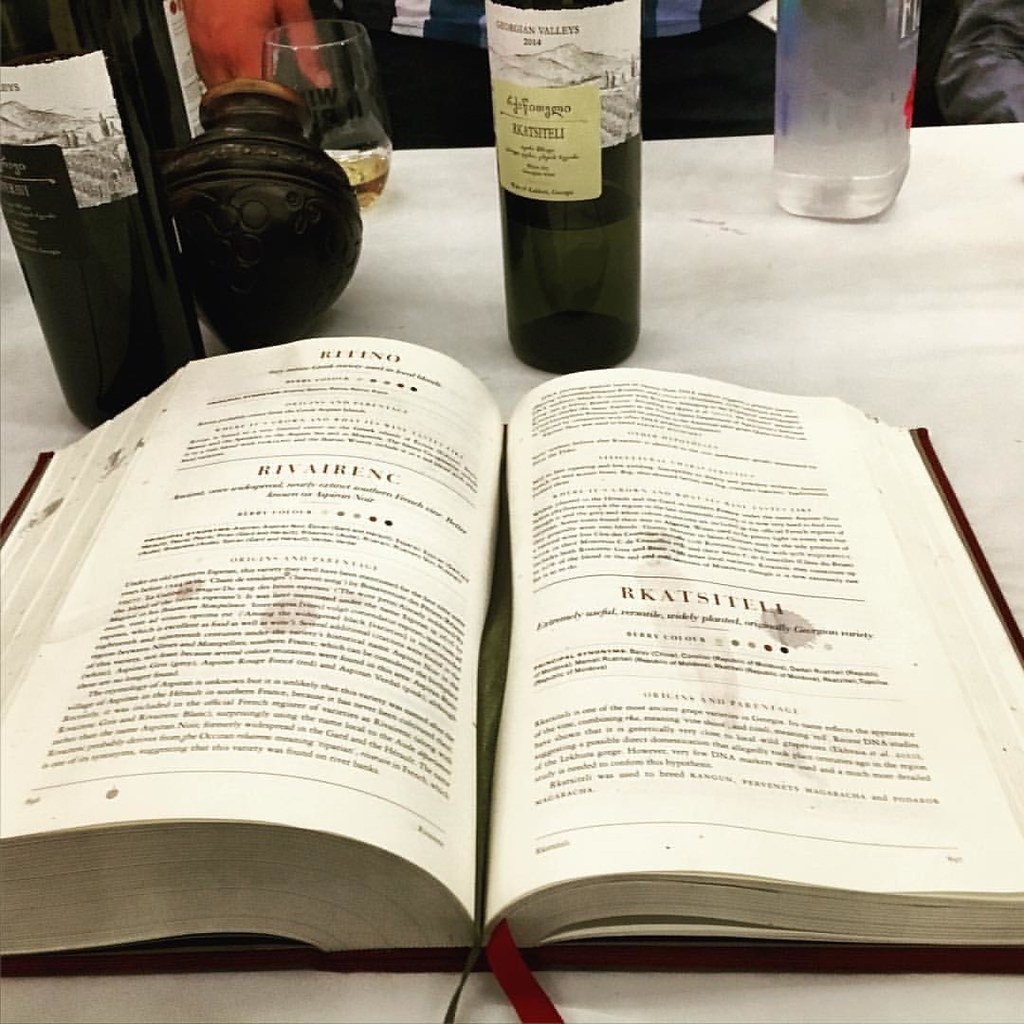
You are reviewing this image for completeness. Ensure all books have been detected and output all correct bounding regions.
[0,335,1024,976]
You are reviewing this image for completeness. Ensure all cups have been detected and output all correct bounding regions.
[261,20,394,212]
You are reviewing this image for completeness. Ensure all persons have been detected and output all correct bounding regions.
[180,0,1022,149]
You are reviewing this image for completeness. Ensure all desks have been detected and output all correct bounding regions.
[0,122,1024,1023]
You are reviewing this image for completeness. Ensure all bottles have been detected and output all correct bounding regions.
[485,0,643,373]
[105,1,200,153]
[775,0,921,221]
[0,0,202,440]
